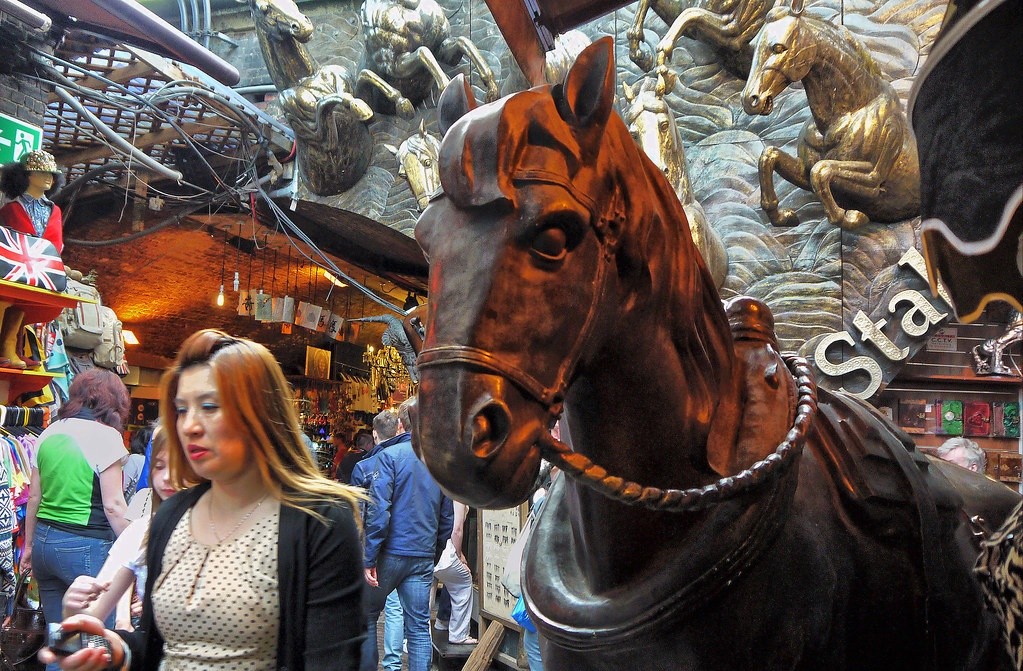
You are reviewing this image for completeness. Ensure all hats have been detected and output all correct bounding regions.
[17,150,63,174]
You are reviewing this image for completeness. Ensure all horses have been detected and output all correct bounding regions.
[618,71,727,294]
[233,1,416,196]
[356,0,500,114]
[384,120,441,211]
[741,1,920,229]
[414,35,1023,671]
[628,0,785,94]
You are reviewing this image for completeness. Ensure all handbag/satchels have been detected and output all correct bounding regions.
[512,595,539,633]
[0,566,45,671]
[0,226,67,291]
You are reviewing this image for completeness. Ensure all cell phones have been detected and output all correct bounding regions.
[46,623,113,664]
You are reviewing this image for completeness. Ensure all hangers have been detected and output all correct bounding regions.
[0,403,51,439]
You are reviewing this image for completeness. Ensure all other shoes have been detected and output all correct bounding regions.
[433,619,450,630]
[450,636,479,645]
[402,639,408,652]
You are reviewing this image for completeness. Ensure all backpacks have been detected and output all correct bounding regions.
[57,276,103,350]
[94,306,125,369]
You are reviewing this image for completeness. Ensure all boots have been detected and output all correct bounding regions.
[19,319,41,368]
[3,307,26,371]
[0,356,10,367]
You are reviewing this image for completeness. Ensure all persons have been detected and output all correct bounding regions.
[63,428,180,629]
[352,411,404,671]
[336,434,373,485]
[0,149,62,250]
[937,437,987,475]
[331,433,346,473]
[434,586,451,630]
[523,466,560,671]
[430,501,481,644]
[19,369,130,671]
[363,396,454,671]
[37,330,366,670]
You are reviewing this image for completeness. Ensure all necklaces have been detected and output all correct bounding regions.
[207,493,271,543]
